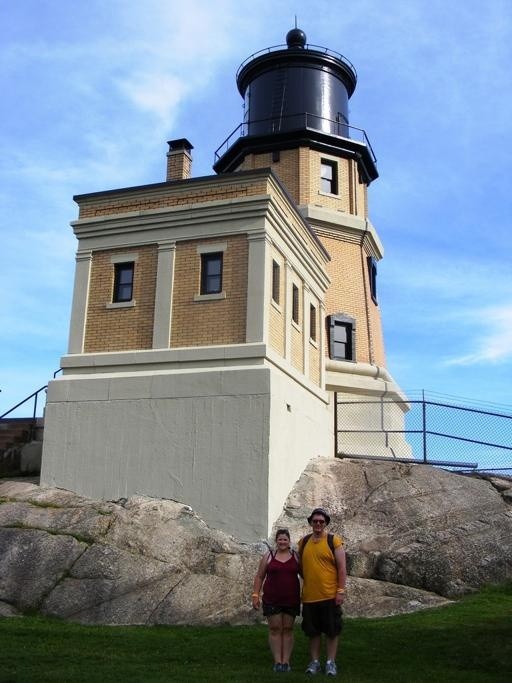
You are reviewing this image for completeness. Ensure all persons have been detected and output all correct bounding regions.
[295,507,348,677]
[253,529,303,672]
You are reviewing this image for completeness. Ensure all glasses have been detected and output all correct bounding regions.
[311,519,325,523]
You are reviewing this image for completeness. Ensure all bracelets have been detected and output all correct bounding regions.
[252,594,259,597]
[336,588,345,593]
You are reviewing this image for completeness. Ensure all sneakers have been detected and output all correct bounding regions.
[325,661,337,677]
[273,662,292,672]
[304,660,322,675]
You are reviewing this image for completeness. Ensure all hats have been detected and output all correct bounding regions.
[307,508,330,525]
